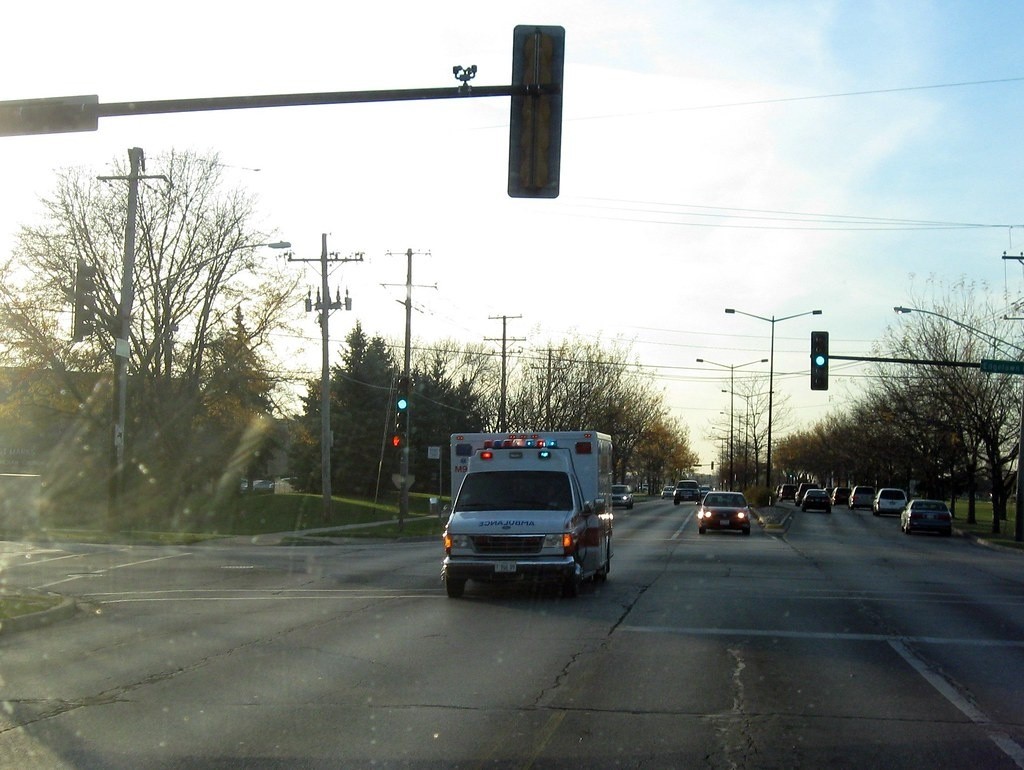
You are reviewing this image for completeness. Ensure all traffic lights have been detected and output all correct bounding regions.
[391,433,407,449]
[811,331,829,391]
[396,377,409,433]
[70,258,98,344]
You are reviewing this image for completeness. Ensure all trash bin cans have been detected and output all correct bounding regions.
[429,497,439,514]
[0,473,42,532]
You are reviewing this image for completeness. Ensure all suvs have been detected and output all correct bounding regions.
[793,483,821,506]
[778,483,799,503]
[673,479,702,506]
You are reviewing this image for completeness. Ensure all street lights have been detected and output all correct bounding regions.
[724,307,823,502]
[107,240,294,532]
[720,389,775,491]
[709,410,761,480]
[696,358,769,494]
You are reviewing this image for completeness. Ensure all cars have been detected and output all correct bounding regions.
[660,486,674,499]
[776,485,781,496]
[608,485,636,509]
[696,490,752,536]
[832,487,854,507]
[824,487,834,496]
[848,486,877,510]
[900,499,953,536]
[872,488,910,517]
[699,486,713,498]
[799,489,833,514]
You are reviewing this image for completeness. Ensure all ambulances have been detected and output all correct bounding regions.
[439,431,616,600]
